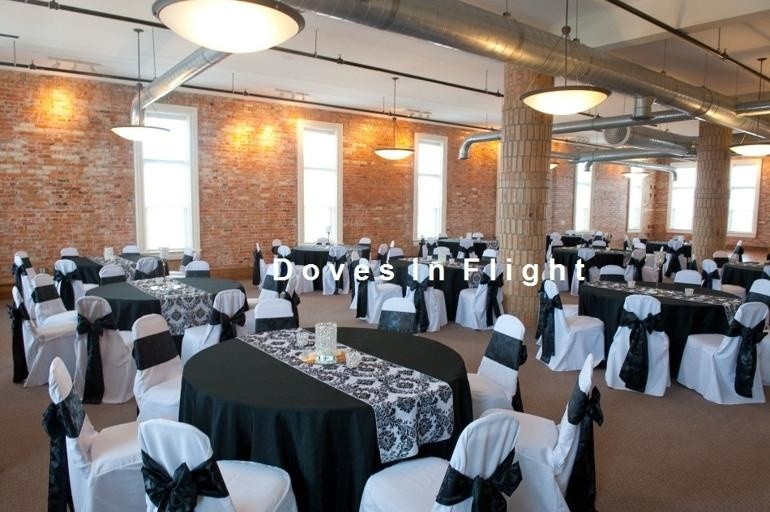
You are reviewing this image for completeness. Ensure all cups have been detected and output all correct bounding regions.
[627,280,635,287]
[295,333,309,347]
[449,258,455,264]
[345,351,361,368]
[314,322,338,365]
[427,256,432,261]
[103,247,114,262]
[685,288,694,296]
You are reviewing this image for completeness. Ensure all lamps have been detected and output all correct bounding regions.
[728,57,770,157]
[372,76,417,161]
[152,0,305,55]
[518,0,610,115]
[110,27,174,142]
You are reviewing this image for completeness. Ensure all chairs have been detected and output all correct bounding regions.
[478,353,607,511]
[359,414,520,511]
[467,314,527,412]
[134,420,299,512]
[43,360,158,512]
[254,236,499,331]
